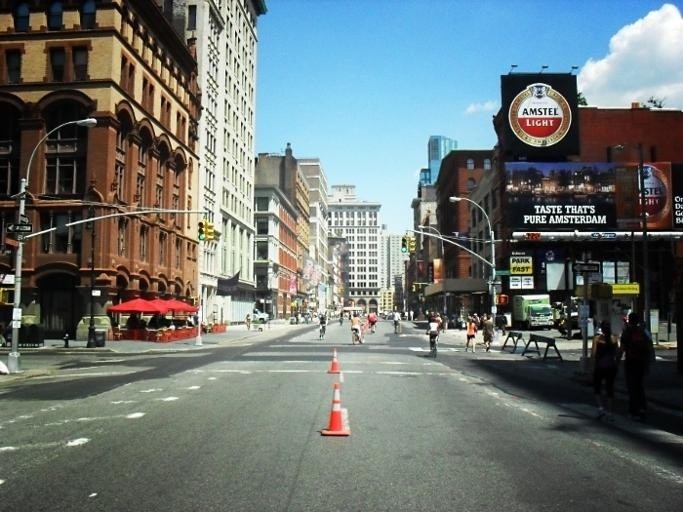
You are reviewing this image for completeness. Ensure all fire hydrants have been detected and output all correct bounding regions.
[62,331,69,348]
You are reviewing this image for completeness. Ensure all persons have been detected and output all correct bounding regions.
[590,319,617,423]
[243,313,250,330]
[317,303,508,356]
[617,311,650,420]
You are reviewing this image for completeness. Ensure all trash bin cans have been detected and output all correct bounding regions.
[96,331,105,347]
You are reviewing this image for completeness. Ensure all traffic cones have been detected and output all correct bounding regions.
[324,346,342,375]
[317,380,351,437]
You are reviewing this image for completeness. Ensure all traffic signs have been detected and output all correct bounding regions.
[4,222,33,234]
[18,214,30,225]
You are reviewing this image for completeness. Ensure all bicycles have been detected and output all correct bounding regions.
[313,312,438,359]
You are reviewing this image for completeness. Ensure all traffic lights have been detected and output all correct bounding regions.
[197,219,206,241]
[408,236,416,253]
[400,236,407,253]
[205,221,214,241]
[495,293,509,305]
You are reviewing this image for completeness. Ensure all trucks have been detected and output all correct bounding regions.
[511,292,555,331]
[228,300,269,324]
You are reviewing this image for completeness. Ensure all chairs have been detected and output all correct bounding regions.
[5,324,44,348]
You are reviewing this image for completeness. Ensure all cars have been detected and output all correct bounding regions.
[553,296,604,341]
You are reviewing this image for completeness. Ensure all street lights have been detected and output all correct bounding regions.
[85,204,98,349]
[4,116,100,376]
[446,194,498,331]
[611,136,653,335]
[416,225,446,278]
